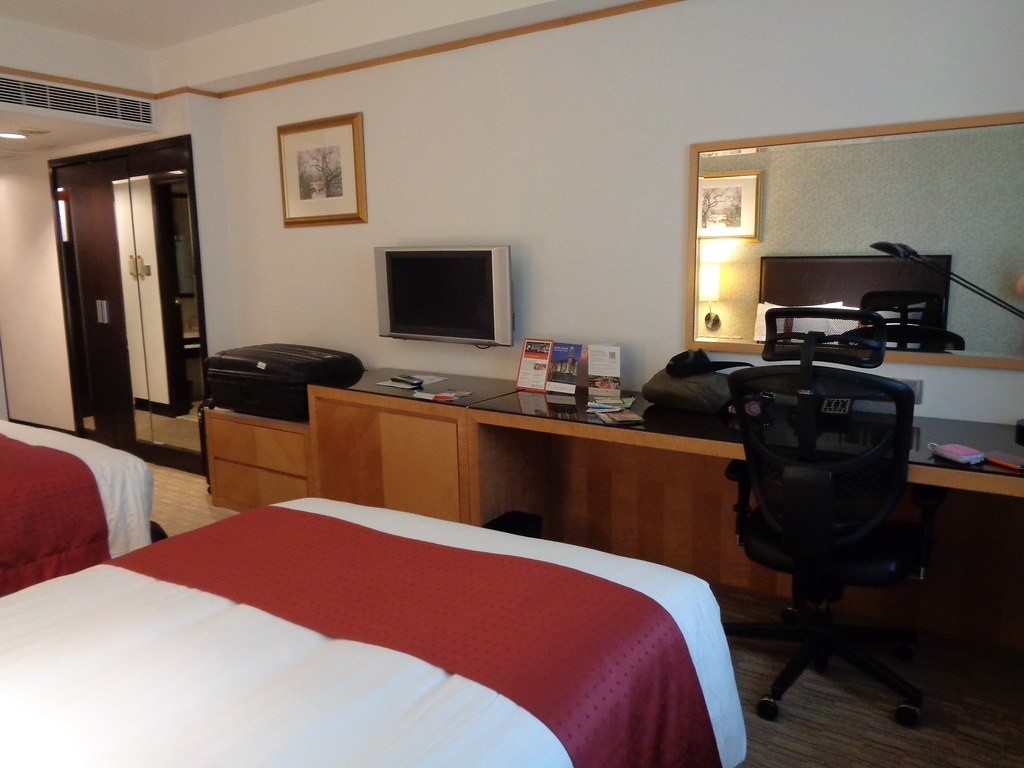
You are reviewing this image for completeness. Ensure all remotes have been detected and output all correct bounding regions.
[391,374,423,386]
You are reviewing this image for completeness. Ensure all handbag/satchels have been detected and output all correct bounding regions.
[642,350,757,413]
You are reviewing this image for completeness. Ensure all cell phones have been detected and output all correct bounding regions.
[984,451,1024,469]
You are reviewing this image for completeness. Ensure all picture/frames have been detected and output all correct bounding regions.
[697,169,765,240]
[276,112,368,229]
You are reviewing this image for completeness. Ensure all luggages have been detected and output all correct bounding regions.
[205,344,367,422]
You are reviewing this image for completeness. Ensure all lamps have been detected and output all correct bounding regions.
[698,264,721,329]
[870,241,1024,322]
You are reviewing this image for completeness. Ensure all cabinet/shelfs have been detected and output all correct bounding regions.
[205,406,307,514]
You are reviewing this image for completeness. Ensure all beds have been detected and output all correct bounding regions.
[756,253,952,345]
[0,497,747,768]
[0,419,153,597]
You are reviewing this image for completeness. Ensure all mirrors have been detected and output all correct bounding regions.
[68,168,203,475]
[684,111,1023,372]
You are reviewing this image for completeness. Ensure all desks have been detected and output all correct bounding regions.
[308,367,1024,655]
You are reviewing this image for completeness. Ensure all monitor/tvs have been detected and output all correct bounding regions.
[374,245,514,348]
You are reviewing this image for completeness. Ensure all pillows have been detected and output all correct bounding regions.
[754,302,926,349]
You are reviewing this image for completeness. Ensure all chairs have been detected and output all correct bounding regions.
[725,308,926,729]
